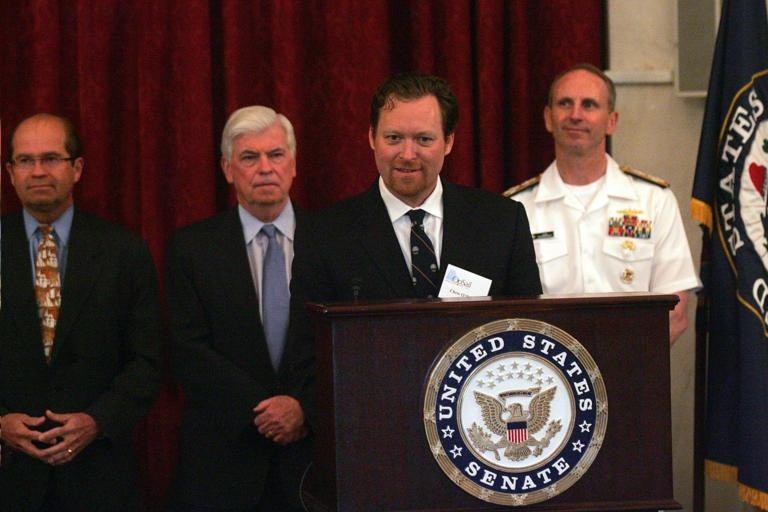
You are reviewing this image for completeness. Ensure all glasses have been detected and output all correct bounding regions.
[7,155,75,168]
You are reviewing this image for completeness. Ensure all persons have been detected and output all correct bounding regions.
[501,66,703,352]
[0,114,162,512]
[164,107,312,512]
[289,74,544,411]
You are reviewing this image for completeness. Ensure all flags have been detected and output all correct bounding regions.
[687,0,768,512]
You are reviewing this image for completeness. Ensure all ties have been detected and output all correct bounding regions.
[405,209,441,300]
[259,224,290,376]
[34,225,63,372]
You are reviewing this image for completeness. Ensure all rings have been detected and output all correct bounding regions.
[67,448,73,456]
